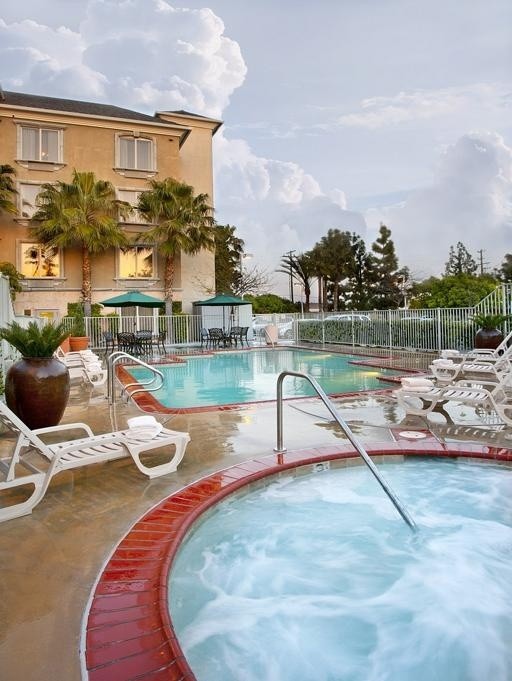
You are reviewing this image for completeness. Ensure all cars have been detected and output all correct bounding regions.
[400,315,433,321]
[324,314,371,322]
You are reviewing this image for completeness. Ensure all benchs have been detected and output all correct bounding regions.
[0,402,191,522]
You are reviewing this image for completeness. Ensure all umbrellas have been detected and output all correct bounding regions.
[99,289,166,333]
[195,293,253,334]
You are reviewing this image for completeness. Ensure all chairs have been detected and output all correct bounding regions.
[52,346,109,394]
[200,327,248,351]
[392,330,512,426]
[103,329,167,356]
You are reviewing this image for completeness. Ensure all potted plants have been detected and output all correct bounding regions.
[0,320,76,429]
[469,311,512,355]
[69,315,88,352]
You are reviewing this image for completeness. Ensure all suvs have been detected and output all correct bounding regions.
[251,316,294,338]
